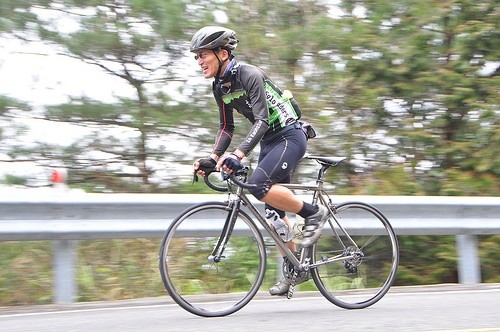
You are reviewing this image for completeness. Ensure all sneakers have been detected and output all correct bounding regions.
[268,272,295,295]
[301,204,328,248]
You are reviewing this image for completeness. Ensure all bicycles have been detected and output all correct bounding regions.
[159,152,399,317]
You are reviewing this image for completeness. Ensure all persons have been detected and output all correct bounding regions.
[190,26,328,295]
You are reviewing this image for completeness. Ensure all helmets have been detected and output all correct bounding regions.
[189,25,239,53]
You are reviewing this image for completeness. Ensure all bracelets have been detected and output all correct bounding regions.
[230,153,240,160]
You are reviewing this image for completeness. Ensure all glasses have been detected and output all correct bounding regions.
[194,50,215,61]
